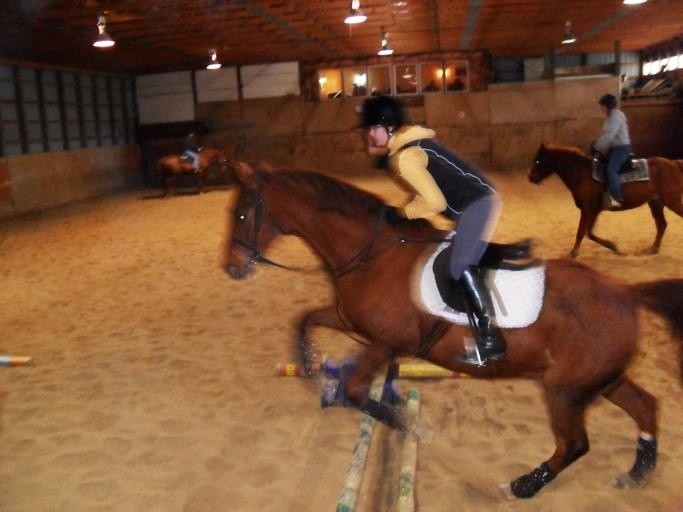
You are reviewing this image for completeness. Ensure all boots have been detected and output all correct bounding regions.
[453,265,508,365]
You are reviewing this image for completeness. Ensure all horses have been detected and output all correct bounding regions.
[219,156,683,502]
[159,148,225,201]
[527,139,683,260]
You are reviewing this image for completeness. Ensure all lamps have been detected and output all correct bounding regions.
[89,14,116,52]
[205,45,222,74]
[375,30,396,58]
[341,0,369,24]
[559,18,577,45]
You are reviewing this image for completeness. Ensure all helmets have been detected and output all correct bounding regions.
[597,93,618,109]
[351,96,412,126]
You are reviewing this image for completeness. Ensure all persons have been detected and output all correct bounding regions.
[174,129,201,175]
[352,89,511,373]
[421,79,438,92]
[589,94,638,210]
[445,77,465,92]
